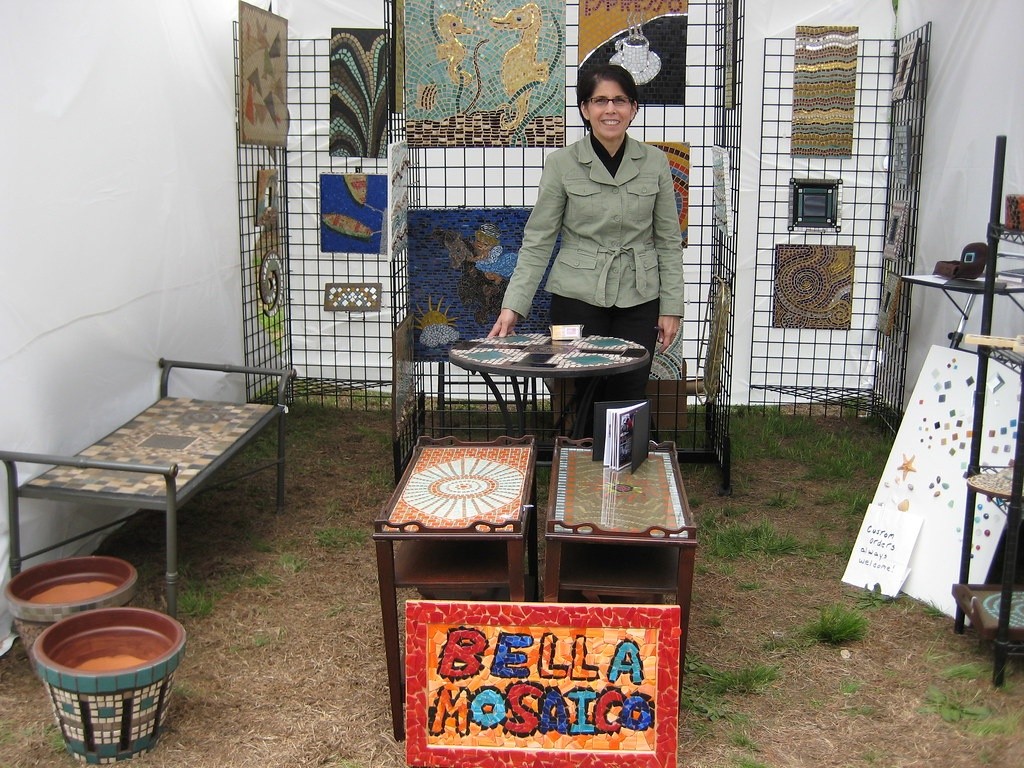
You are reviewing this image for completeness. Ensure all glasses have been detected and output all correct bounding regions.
[586,94,634,106]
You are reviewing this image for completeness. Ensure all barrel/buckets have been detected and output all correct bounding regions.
[32,606,189,764]
[4,556,139,686]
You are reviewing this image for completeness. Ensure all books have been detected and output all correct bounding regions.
[592,401,652,474]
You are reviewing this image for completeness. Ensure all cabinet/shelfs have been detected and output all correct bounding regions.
[949,134,1024,686]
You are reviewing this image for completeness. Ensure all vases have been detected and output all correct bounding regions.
[29,607,187,766]
[2,554,139,686]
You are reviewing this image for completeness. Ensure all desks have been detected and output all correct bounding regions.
[542,436,698,674]
[447,332,650,465]
[370,435,537,743]
[0,355,296,621]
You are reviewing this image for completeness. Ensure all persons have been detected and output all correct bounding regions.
[487,65,686,442]
